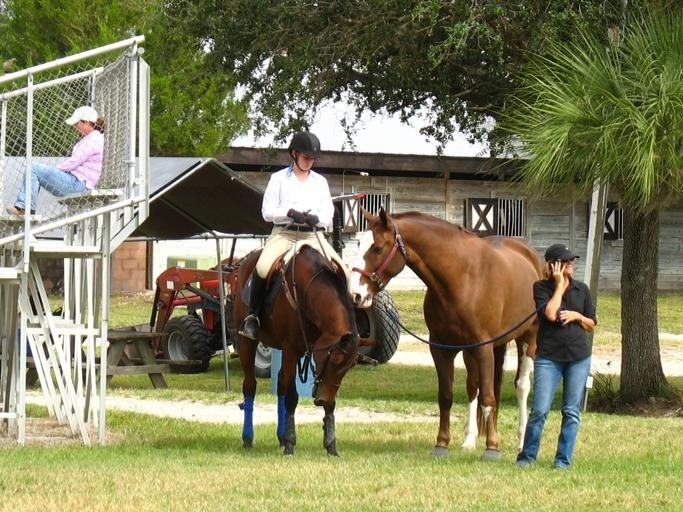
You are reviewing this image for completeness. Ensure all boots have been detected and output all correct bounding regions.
[343,292,360,338]
[238,267,267,342]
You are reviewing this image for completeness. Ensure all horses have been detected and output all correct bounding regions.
[231,243,381,461]
[346,205,550,461]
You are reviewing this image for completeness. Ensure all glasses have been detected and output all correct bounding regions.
[560,258,576,263]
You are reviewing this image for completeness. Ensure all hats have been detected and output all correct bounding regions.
[544,243,580,262]
[64,105,98,126]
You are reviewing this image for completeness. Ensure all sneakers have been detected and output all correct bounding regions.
[5,205,35,217]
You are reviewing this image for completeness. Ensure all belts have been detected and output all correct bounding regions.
[281,224,323,234]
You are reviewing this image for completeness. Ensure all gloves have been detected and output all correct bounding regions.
[286,208,320,225]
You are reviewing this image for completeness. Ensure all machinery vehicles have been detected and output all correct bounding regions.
[108,257,400,373]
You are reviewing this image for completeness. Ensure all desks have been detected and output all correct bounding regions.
[98,328,169,390]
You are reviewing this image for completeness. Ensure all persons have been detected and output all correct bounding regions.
[0,289,54,389]
[242,131,350,341]
[515,243,597,471]
[6,106,104,218]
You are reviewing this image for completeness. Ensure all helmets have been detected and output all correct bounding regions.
[288,131,322,157]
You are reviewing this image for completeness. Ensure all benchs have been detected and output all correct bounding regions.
[7,352,203,390]
[0,187,123,278]
